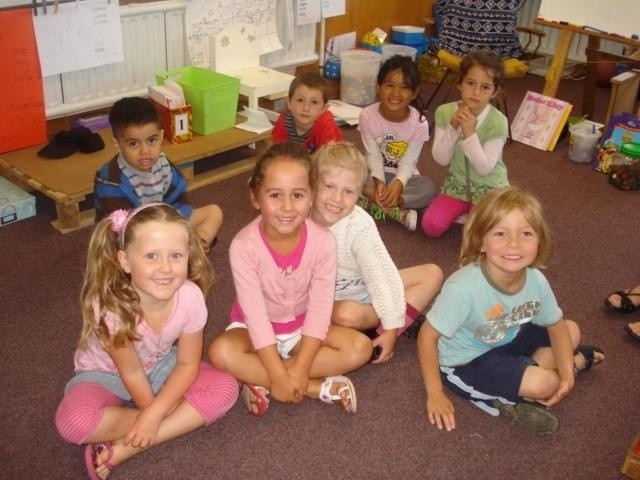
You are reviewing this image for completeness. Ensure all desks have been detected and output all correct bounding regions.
[0,114,278,237]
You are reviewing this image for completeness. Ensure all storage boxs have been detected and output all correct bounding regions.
[391,24,426,46]
[148,104,194,144]
[0,174,37,228]
[149,67,241,136]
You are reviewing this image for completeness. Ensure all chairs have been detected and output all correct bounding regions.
[210,19,299,121]
[422,1,546,145]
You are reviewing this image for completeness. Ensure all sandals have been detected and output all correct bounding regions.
[603,284,640,312]
[320,375,358,414]
[574,346,606,378]
[494,397,558,438]
[625,318,640,340]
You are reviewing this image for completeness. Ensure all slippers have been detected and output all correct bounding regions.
[85,441,116,480]
[241,383,270,418]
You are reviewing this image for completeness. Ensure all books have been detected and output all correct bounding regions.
[511,90,572,152]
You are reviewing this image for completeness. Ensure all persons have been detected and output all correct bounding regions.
[421,50,508,239]
[207,141,374,417]
[309,141,444,365]
[94,97,224,255]
[54,202,239,480]
[272,72,342,152]
[417,188,604,432]
[605,283,640,343]
[357,54,439,232]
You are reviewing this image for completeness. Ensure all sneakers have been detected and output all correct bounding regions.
[387,206,418,232]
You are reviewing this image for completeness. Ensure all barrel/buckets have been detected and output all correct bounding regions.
[568,124,602,164]
[381,44,417,63]
[339,50,382,107]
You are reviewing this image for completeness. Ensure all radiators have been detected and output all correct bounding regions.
[31,0,190,124]
